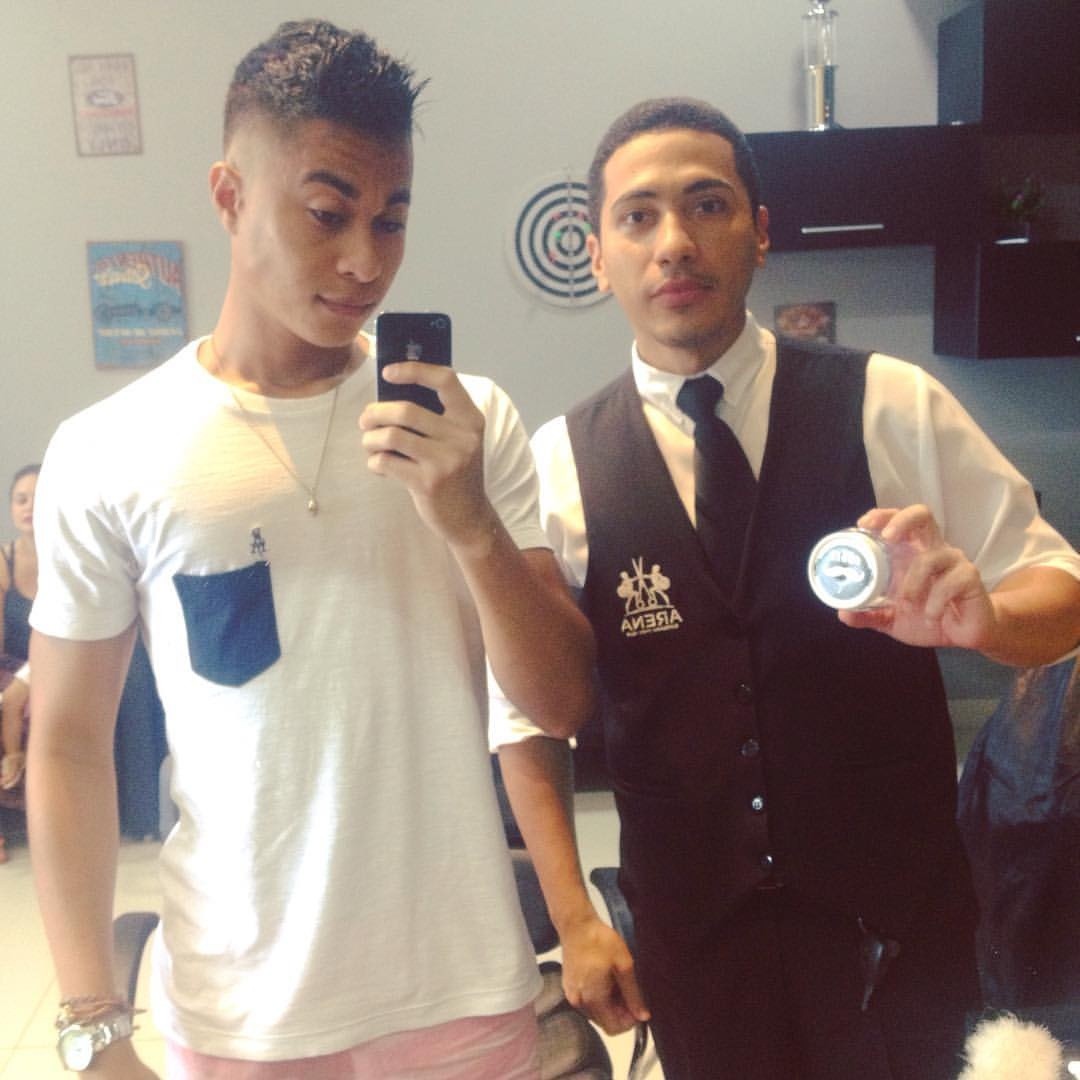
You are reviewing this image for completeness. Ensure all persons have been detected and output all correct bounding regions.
[1,659,30,790]
[483,97,1080,1079]
[24,18,599,1080]
[0,463,40,856]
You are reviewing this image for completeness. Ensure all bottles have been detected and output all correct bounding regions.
[809,526,921,613]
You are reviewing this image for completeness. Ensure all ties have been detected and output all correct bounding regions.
[675,374,756,604]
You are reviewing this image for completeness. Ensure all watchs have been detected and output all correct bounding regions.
[57,1014,131,1070]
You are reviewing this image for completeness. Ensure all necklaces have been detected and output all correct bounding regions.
[211,336,346,516]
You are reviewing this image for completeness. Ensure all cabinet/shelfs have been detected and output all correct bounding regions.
[747,0,1080,359]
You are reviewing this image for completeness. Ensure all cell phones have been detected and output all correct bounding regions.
[378,312,452,460]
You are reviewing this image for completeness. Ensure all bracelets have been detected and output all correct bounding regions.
[53,995,146,1027]
